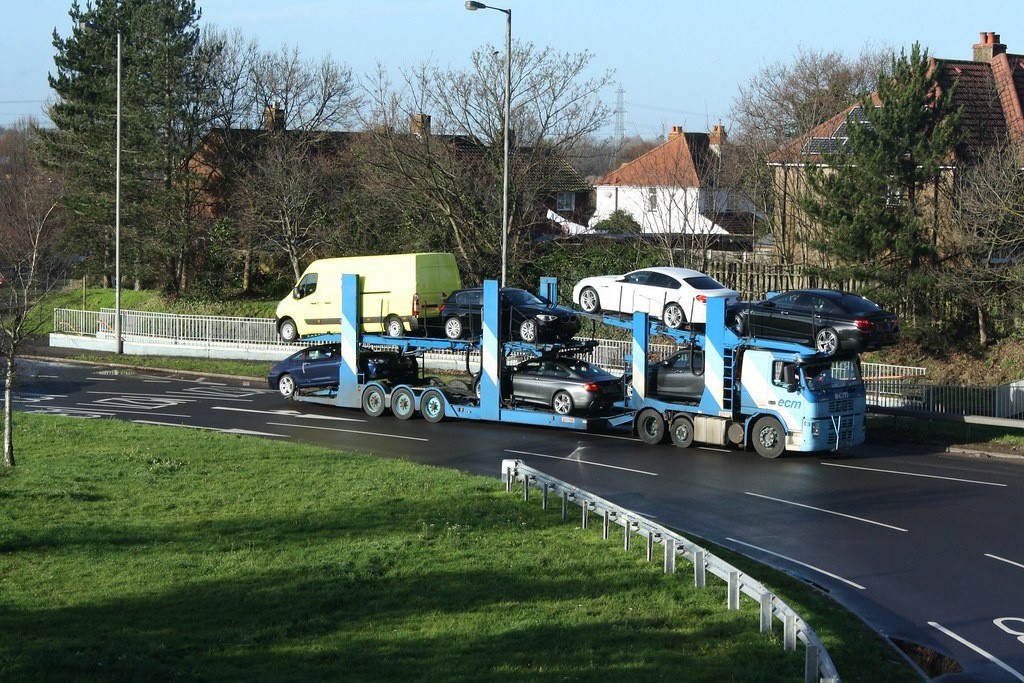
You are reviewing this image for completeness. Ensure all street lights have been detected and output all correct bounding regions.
[77,18,125,355]
[461,2,513,291]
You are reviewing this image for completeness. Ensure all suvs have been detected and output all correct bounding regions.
[438,285,580,344]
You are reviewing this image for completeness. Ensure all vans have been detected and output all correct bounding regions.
[273,251,463,342]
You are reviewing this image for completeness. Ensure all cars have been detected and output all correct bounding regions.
[571,266,742,332]
[727,288,901,355]
[266,341,419,396]
[618,348,703,400]
[469,356,623,416]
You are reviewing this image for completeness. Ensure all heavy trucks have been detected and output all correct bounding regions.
[289,273,869,458]
